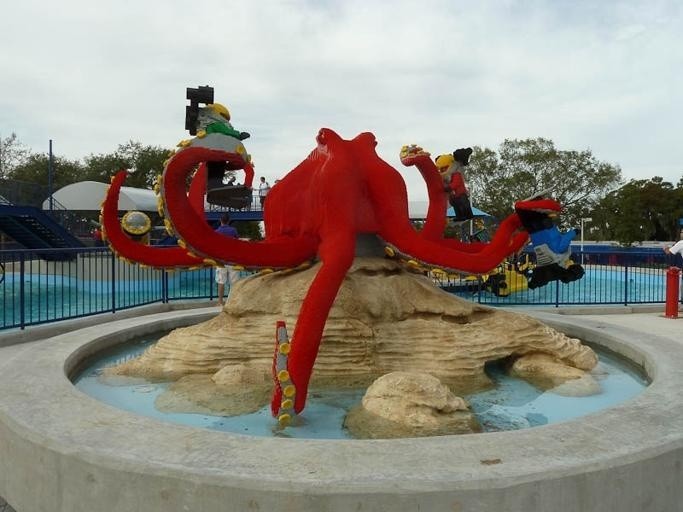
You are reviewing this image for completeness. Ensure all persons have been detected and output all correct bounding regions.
[227,176,235,213]
[212,212,238,305]
[257,177,269,212]
[662,229,682,304]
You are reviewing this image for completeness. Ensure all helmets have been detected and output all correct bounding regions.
[436,155,457,176]
[207,104,230,123]
